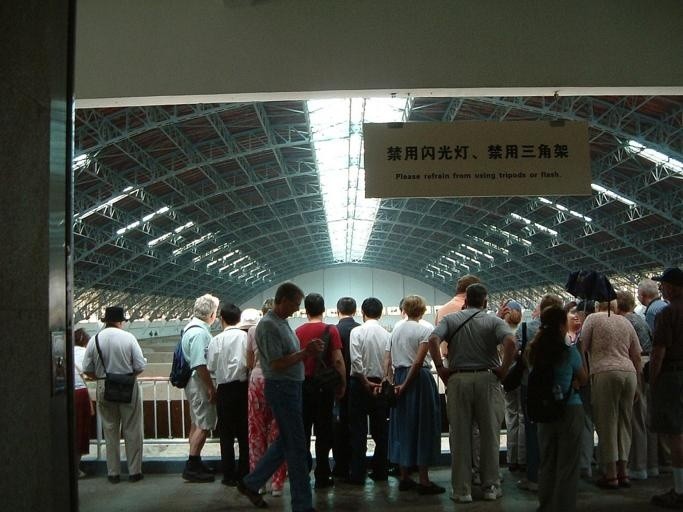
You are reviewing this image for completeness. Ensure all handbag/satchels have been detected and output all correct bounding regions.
[104,373,136,403]
[315,366,343,393]
[504,360,524,391]
[526,342,575,422]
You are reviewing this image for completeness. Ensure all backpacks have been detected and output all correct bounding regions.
[170,326,208,389]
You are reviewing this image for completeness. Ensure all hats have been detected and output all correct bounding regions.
[101,307,128,322]
[570,300,596,314]
[651,268,683,281]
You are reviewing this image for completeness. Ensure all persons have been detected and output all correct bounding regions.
[73,326,96,479]
[169,266,682,511]
[81,306,146,484]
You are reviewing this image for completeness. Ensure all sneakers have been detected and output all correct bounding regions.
[108,475,120,484]
[628,464,682,510]
[129,473,144,482]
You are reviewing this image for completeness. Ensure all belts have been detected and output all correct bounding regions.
[449,369,492,373]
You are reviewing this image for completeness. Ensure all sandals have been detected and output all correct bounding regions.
[619,476,630,487]
[597,478,618,488]
